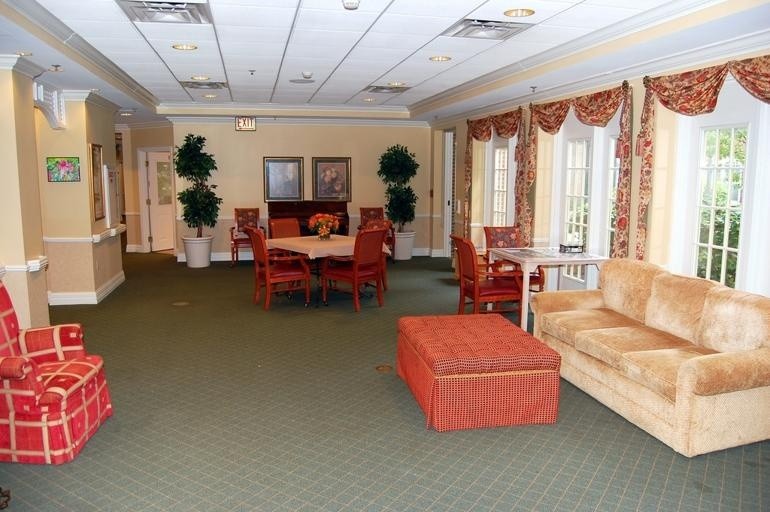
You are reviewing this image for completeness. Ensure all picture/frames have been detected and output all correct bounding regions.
[89,143,106,223]
[312,157,351,202]
[263,157,304,203]
[47,157,80,182]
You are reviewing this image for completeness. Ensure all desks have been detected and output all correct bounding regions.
[268,201,350,239]
[487,246,612,332]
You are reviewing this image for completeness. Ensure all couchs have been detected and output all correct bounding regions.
[530,257,770,458]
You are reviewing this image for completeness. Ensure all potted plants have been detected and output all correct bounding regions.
[377,142,421,261]
[173,133,223,268]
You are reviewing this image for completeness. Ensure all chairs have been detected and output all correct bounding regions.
[449,234,522,325]
[230,207,396,312]
[483,227,545,292]
[0,280,113,464]
